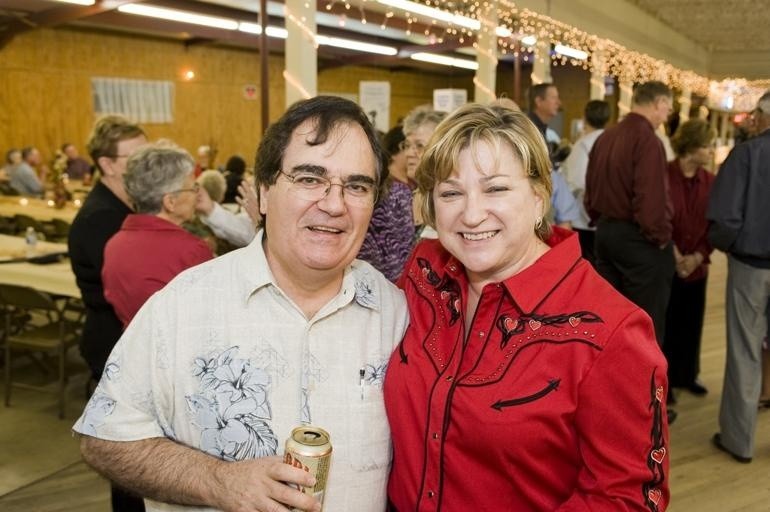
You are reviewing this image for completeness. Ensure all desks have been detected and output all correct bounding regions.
[0,235,85,356]
[0,196,81,225]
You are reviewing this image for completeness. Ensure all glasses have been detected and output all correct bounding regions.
[696,141,718,150]
[104,154,129,160]
[398,139,427,150]
[174,183,201,195]
[276,167,379,208]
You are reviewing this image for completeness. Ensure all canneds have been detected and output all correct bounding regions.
[283,424,334,512]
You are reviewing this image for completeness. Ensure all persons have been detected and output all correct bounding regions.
[61,142,92,180]
[68,112,147,512]
[193,142,216,178]
[9,146,49,198]
[70,93,413,512]
[664,117,717,405]
[384,95,670,512]
[702,88,770,463]
[525,83,560,175]
[196,178,263,249]
[220,155,250,204]
[355,125,416,286]
[403,103,450,239]
[558,100,612,214]
[0,149,20,182]
[101,138,214,331]
[197,169,226,206]
[583,79,679,430]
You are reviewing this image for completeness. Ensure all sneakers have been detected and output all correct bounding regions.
[664,389,678,405]
[686,380,710,397]
[666,408,681,423]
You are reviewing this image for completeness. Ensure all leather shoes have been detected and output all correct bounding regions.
[711,433,751,462]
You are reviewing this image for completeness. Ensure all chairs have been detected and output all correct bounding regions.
[0,283,81,419]
[50,217,70,242]
[13,213,49,241]
